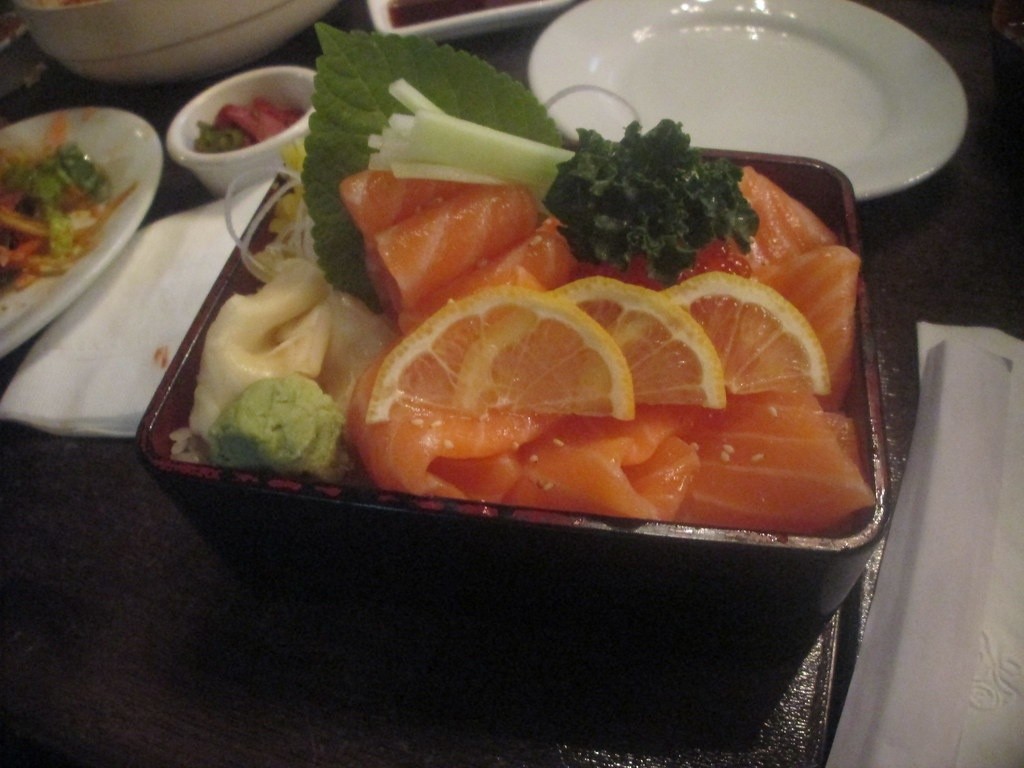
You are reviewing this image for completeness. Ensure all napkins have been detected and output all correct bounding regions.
[0,175,275,442]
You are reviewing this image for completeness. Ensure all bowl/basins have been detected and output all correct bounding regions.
[137,139,892,747]
[16,2,346,87]
[165,65,318,201]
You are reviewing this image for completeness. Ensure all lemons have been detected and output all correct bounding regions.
[365,278,836,426]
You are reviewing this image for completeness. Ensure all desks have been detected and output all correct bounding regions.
[0,0,1023,768]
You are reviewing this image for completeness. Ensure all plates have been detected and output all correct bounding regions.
[365,0,577,44]
[2,107,164,361]
[529,0,970,202]
